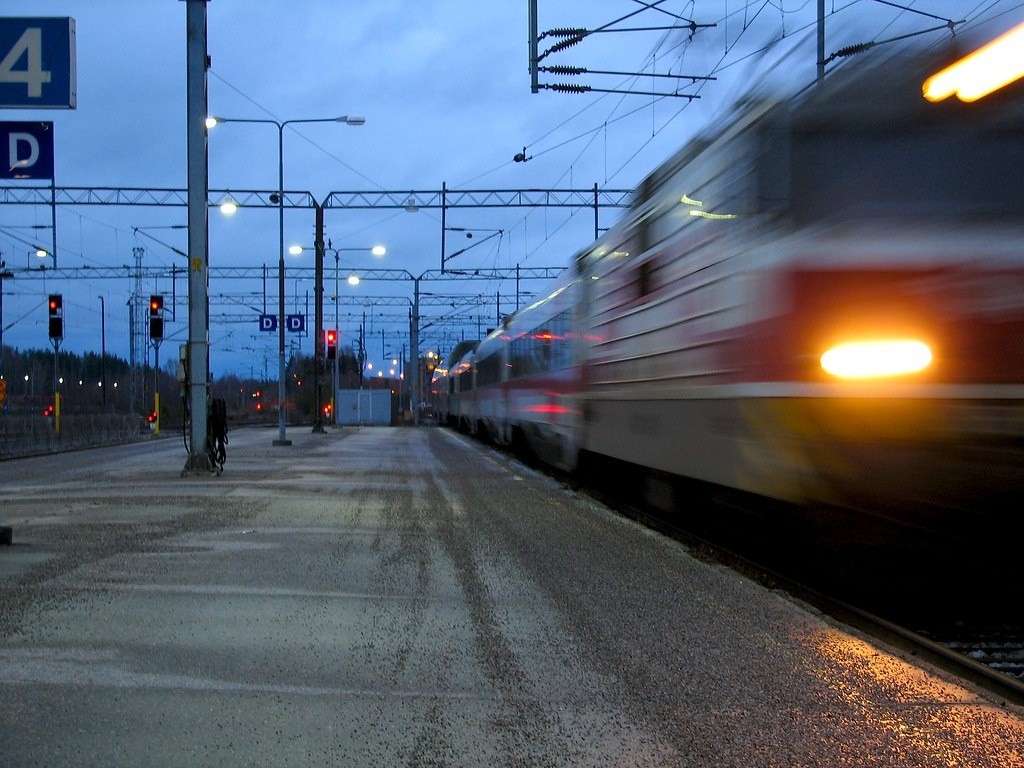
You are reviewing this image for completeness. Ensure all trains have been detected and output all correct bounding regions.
[426,35,1024,571]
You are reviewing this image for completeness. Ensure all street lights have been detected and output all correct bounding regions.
[289,244,385,433]
[98,296,105,407]
[208,114,367,441]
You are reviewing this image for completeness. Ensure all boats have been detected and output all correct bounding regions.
[256,390,261,398]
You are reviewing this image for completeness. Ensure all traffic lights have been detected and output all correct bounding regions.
[147,412,158,421]
[44,406,53,417]
[49,295,63,339]
[257,404,261,410]
[323,404,331,413]
[149,296,163,340]
[327,330,337,359]
